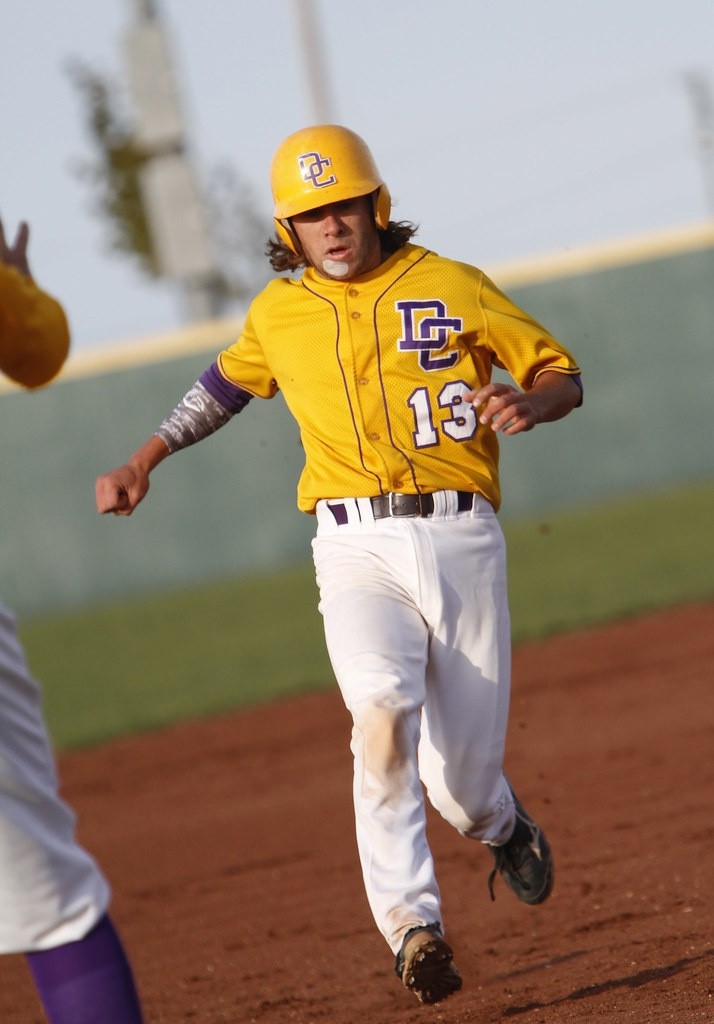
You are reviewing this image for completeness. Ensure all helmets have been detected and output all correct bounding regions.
[271,124,384,219]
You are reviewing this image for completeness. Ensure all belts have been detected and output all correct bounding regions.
[329,489,473,525]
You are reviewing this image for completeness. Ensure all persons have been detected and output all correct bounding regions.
[0,222,144,1024]
[95,122,587,1002]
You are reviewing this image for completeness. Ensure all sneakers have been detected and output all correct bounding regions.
[487,787,553,907]
[396,926,462,1002]
